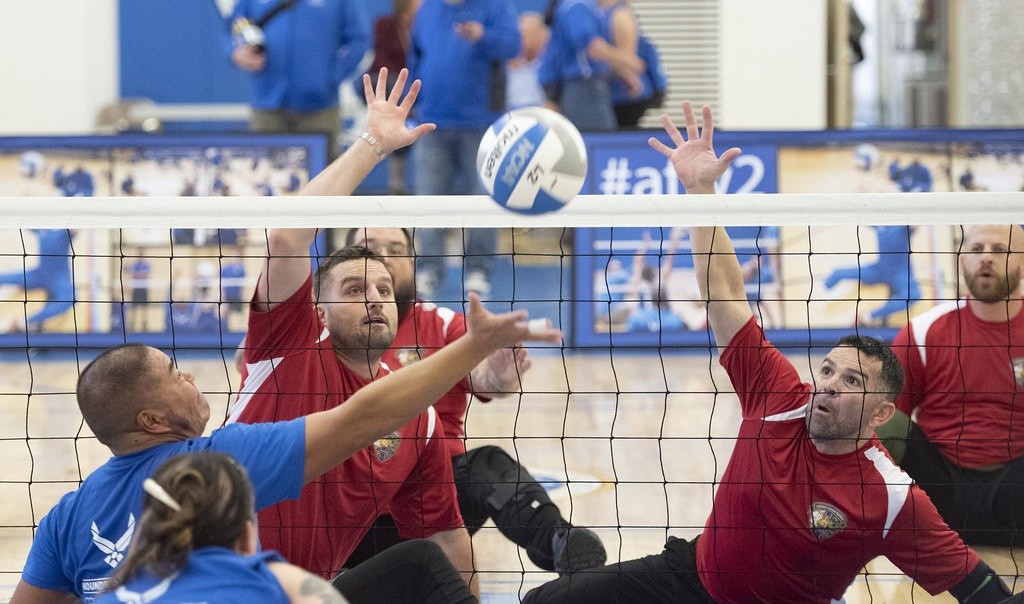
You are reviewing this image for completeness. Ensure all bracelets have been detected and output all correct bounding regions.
[359,132,386,160]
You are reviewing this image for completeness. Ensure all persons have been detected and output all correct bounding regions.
[216,0,664,296]
[519,101,1015,604]
[11,67,607,604]
[875,224,1024,547]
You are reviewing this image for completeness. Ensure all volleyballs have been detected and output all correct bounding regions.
[474,105,590,217]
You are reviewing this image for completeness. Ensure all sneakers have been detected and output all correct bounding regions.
[552,526,608,580]
